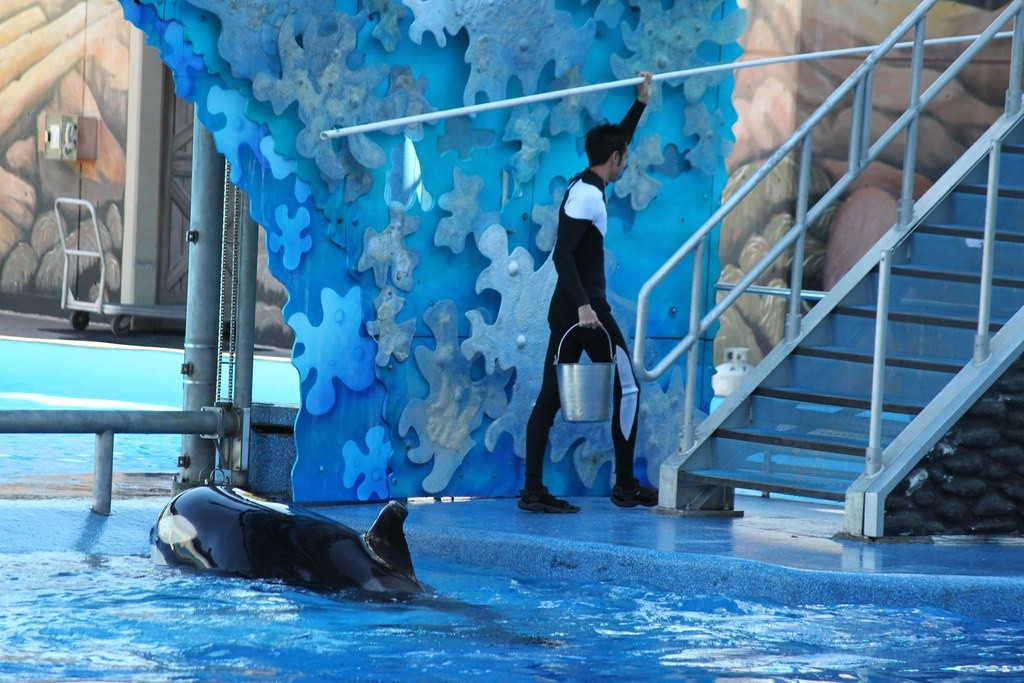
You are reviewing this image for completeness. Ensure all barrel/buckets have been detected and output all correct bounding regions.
[552,320,616,426]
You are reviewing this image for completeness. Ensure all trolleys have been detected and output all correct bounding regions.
[54,197,231,339]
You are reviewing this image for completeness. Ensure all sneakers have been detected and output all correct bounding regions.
[518,487,581,513]
[610,478,658,508]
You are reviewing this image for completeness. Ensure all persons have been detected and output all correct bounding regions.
[515,70,658,515]
